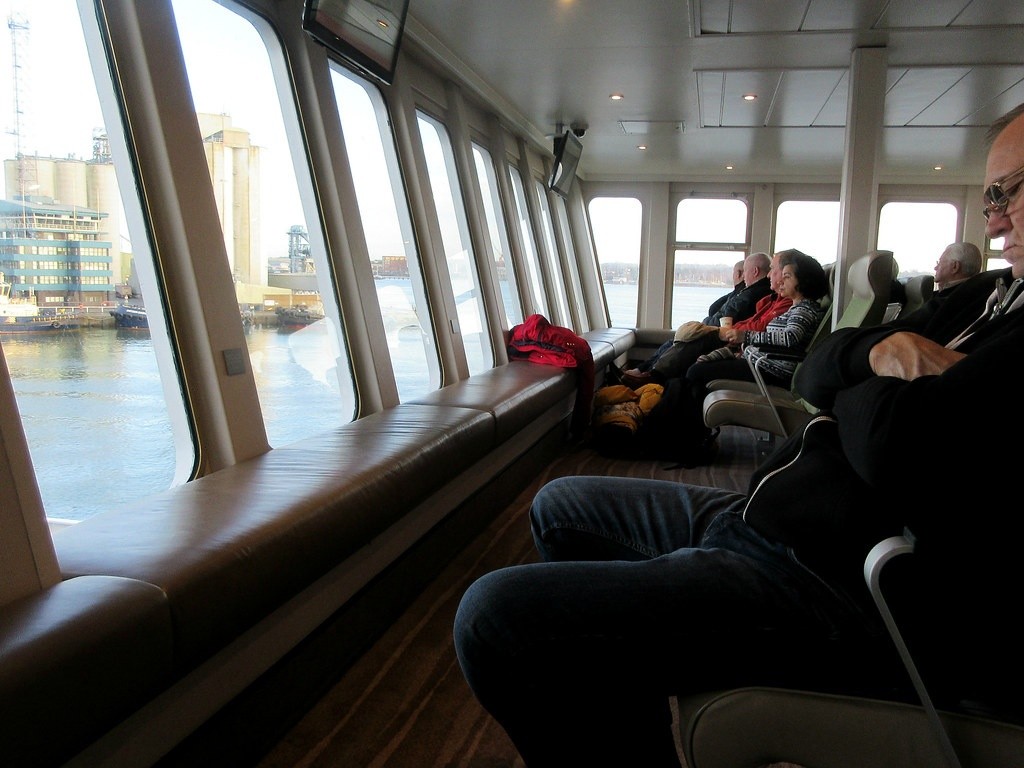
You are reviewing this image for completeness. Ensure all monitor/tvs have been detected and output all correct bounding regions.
[301,0,409,86]
[547,130,583,201]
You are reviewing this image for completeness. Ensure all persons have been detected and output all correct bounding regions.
[934,240,982,293]
[453,102,1024,768]
[606,250,830,444]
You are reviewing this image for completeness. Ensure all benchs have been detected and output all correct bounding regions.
[46,402,499,725]
[581,327,636,378]
[402,360,578,480]
[0,574,174,768]
[584,340,615,393]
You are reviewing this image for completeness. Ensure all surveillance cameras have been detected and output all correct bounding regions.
[573,129,586,139]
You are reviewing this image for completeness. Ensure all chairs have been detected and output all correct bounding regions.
[702,250,935,456]
[678,526,1024,768]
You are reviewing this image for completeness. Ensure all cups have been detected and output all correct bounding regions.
[720,317,732,329]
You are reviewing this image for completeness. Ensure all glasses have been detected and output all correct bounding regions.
[981,167,1024,224]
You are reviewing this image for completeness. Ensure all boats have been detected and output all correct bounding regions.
[241,302,326,326]
[110,302,149,329]
